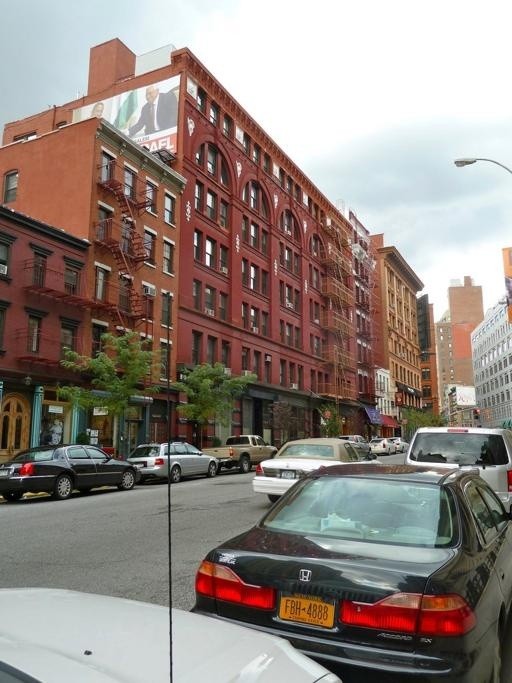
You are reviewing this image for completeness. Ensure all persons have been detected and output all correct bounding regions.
[127,86,177,137]
[40,424,52,447]
[92,103,104,117]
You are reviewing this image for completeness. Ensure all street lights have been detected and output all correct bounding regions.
[453,156,511,176]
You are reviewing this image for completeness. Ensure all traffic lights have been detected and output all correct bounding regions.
[473,409,480,419]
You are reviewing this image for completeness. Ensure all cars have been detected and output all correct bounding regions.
[251,437,371,507]
[127,440,219,487]
[391,437,408,455]
[0,588,345,682]
[182,463,512,683]
[369,438,396,456]
[0,443,141,502]
[339,433,371,457]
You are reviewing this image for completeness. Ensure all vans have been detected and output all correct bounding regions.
[406,427,512,517]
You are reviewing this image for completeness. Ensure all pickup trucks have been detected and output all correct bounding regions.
[200,435,281,475]
